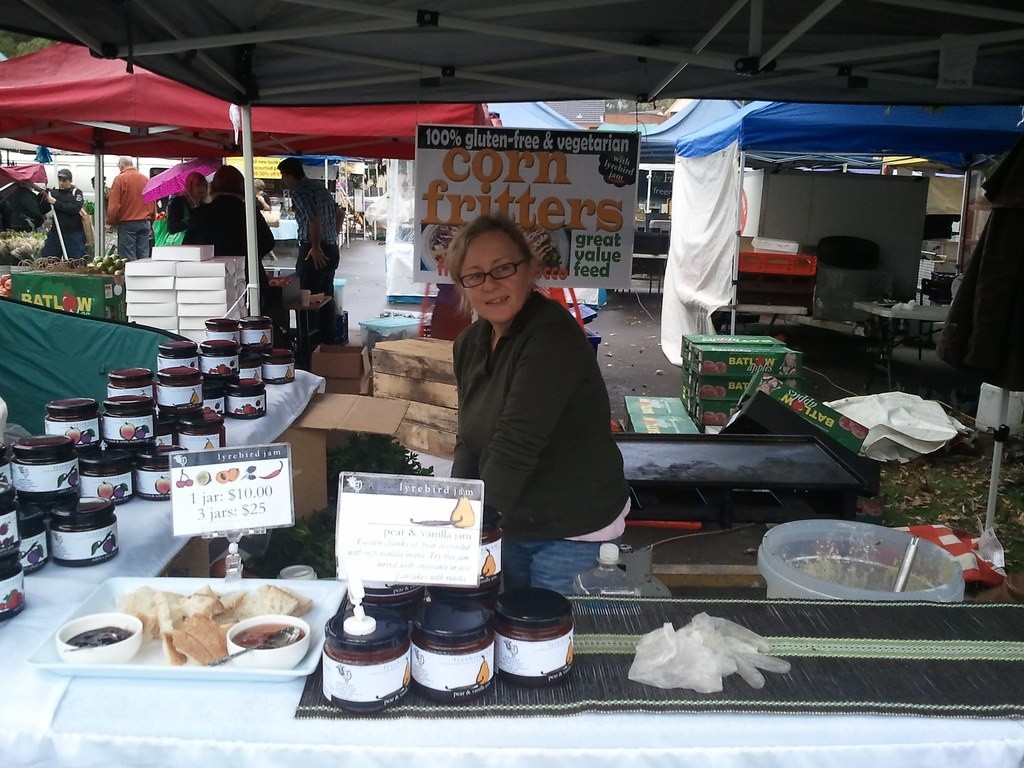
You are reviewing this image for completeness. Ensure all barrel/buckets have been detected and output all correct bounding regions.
[757,519,966,604]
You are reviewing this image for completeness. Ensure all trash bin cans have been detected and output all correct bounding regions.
[815,236,881,322]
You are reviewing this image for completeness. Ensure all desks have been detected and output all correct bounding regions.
[0,370,1024,767]
[269,220,299,240]
[617,231,671,293]
[717,305,807,335]
[853,301,951,392]
[282,295,332,366]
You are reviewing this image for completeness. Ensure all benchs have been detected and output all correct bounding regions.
[777,314,903,342]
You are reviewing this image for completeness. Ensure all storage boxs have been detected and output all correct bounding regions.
[358,316,428,356]
[623,335,881,497]
[332,278,350,347]
[273,392,412,520]
[125,245,246,348]
[312,338,459,461]
[738,237,817,276]
[568,304,602,359]
[10,270,126,323]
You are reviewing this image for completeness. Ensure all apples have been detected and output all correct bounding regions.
[702,361,728,424]
[87,254,130,276]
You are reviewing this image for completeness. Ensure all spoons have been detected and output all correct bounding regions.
[62,633,117,654]
[207,626,303,667]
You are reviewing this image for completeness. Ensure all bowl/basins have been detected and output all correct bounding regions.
[421,225,441,271]
[56,613,143,665]
[550,226,570,267]
[226,614,310,670]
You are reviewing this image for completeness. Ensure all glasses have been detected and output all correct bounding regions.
[457,257,527,288]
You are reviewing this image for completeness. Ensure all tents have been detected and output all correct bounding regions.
[640,99,755,213]
[0,164,68,261]
[269,154,383,248]
[385,102,608,309]
[660,101,1024,368]
[0,39,489,318]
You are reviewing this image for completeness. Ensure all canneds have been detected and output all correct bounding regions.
[0,316,296,623]
[323,504,574,713]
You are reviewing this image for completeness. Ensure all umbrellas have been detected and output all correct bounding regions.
[142,158,223,208]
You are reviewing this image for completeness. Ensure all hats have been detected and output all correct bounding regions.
[58,169,72,180]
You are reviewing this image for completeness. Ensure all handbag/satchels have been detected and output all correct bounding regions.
[72,188,95,244]
[152,200,190,247]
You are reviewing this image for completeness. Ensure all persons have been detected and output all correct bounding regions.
[448,212,633,596]
[26,169,87,269]
[277,157,345,347]
[181,164,275,314]
[253,178,277,265]
[167,171,208,235]
[105,157,156,261]
[91,176,111,208]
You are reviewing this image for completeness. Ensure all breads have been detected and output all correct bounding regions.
[118,586,314,666]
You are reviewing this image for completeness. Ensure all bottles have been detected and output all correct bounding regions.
[572,542,641,616]
